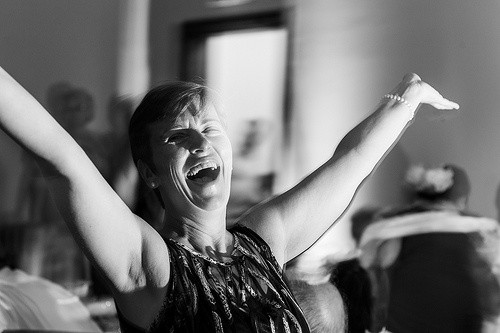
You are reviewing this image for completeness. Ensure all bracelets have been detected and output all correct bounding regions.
[382,93,416,122]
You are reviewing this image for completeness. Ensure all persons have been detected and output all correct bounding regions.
[284,274,346,333]
[0,80,164,333]
[0,62,461,333]
[235,115,271,179]
[326,163,500,333]
